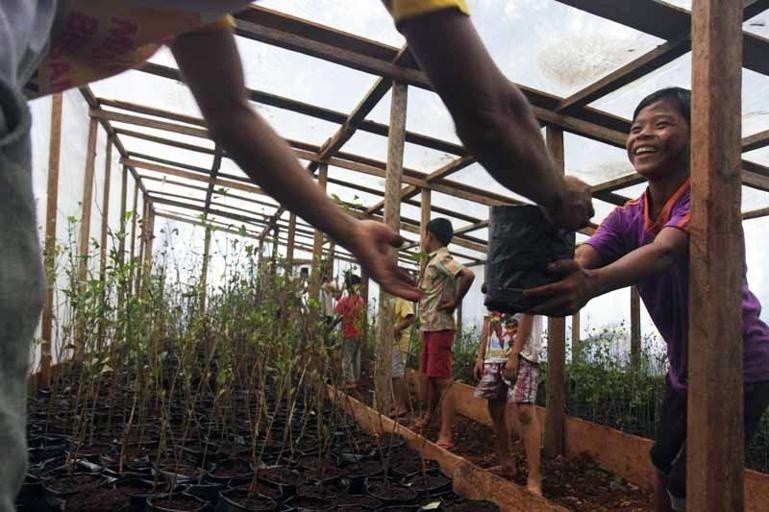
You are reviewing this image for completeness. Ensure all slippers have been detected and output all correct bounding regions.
[435,437,459,450]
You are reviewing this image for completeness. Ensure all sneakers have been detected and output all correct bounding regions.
[389,404,405,416]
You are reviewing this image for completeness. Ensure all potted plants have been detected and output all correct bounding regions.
[366,291,769,474]
[13,167,500,510]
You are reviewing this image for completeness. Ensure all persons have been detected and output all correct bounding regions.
[388,297,416,418]
[319,264,344,361]
[1,1,595,511]
[334,273,366,389]
[406,217,474,450]
[480,86,769,511]
[472,296,543,496]
